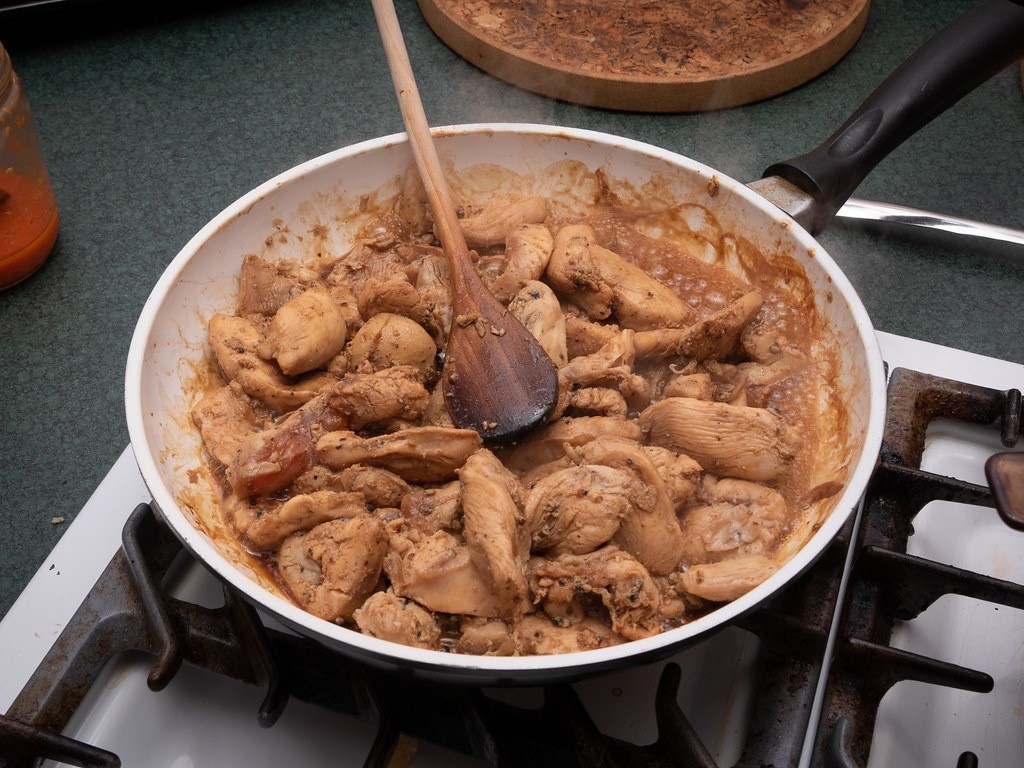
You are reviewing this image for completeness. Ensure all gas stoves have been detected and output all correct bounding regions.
[0,326,1024,768]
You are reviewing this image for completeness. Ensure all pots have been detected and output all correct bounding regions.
[123,0,1024,671]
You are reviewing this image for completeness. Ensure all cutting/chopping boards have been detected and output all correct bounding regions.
[422,0,871,112]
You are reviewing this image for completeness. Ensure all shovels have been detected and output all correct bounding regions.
[371,0,560,444]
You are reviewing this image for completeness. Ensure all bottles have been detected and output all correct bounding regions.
[0,47,60,288]
